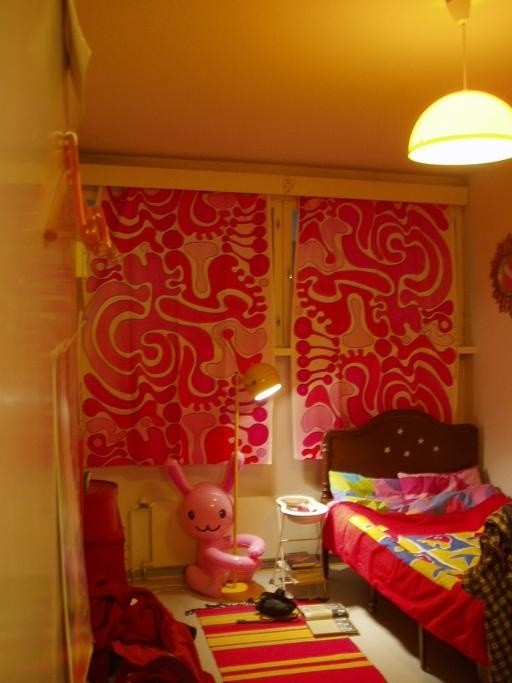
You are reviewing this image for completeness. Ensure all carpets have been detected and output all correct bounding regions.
[191,601,387,683]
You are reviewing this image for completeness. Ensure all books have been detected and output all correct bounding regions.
[278,550,328,586]
[297,601,360,639]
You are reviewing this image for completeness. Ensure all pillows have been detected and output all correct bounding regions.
[329,463,482,508]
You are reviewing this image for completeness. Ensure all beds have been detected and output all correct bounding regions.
[322,408,510,682]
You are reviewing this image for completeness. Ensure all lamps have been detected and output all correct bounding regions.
[218,364,283,601]
[406,0,512,166]
